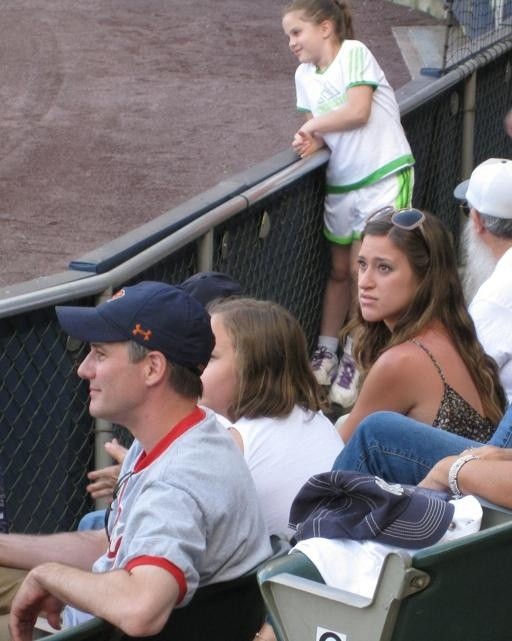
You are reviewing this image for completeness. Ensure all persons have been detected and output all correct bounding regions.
[1,280,273,641]
[332,407,512,507]
[60,271,346,628]
[454,157,512,402]
[336,205,506,445]
[282,2,418,412]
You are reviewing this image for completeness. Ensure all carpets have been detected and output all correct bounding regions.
[391,25,467,81]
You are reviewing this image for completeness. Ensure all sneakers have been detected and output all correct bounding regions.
[309,350,338,386]
[328,352,363,409]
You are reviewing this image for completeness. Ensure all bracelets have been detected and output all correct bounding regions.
[448,454,475,495]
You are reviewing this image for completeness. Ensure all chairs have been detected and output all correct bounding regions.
[32,611,128,641]
[129,527,292,641]
[257,498,512,641]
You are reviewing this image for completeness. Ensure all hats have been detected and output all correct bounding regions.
[288,466,455,551]
[174,271,243,305]
[453,158,512,220]
[53,280,216,378]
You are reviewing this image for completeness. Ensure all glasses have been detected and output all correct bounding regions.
[104,472,135,546]
[459,202,472,218]
[366,206,429,254]
